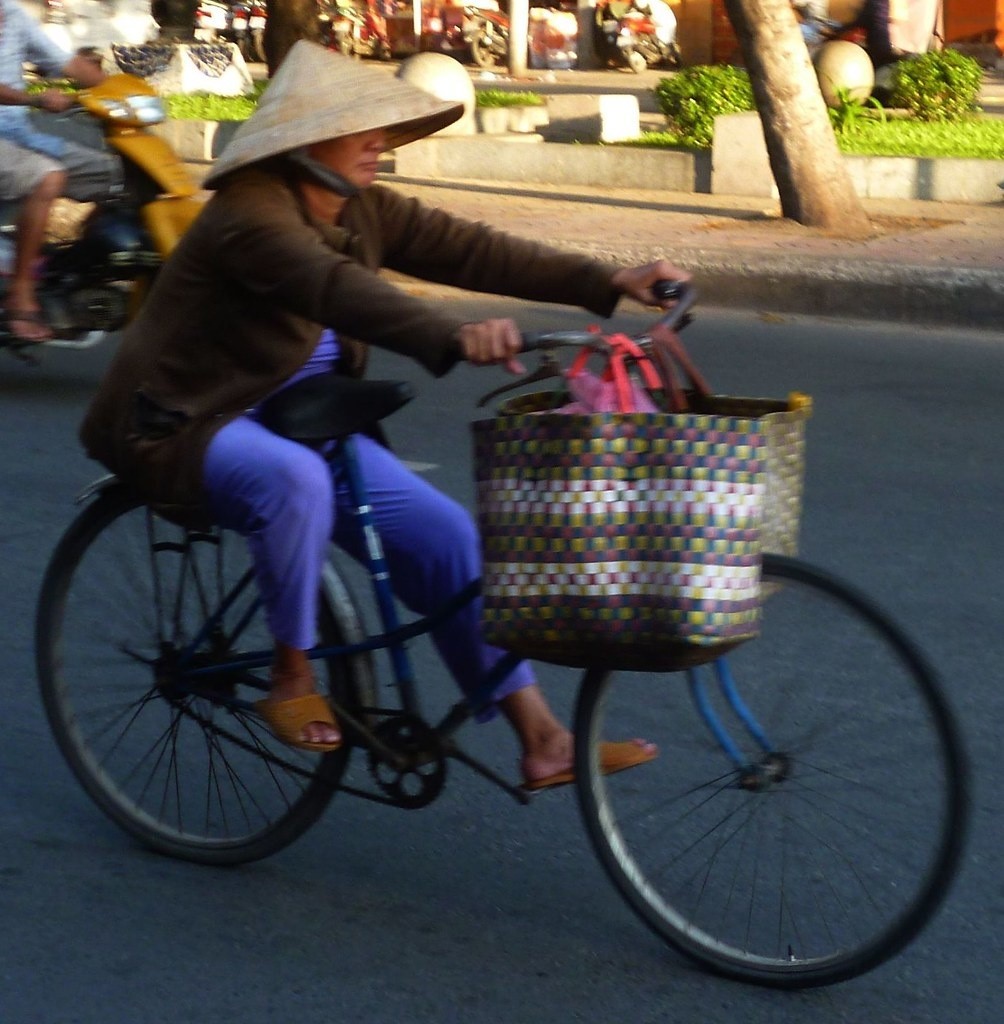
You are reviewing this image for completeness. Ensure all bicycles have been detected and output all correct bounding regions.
[31,278,976,991]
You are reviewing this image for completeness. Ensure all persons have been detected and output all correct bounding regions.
[76,38,692,789]
[0,0,105,341]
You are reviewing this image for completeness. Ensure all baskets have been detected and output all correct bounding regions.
[469,323,811,673]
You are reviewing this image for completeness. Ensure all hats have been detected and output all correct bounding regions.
[201,41,465,190]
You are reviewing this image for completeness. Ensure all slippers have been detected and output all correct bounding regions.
[0,308,54,342]
[254,693,340,751]
[521,740,659,790]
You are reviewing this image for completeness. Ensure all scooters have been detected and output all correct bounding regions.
[192,0,682,77]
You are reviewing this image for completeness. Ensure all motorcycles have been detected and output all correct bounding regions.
[0,73,208,350]
